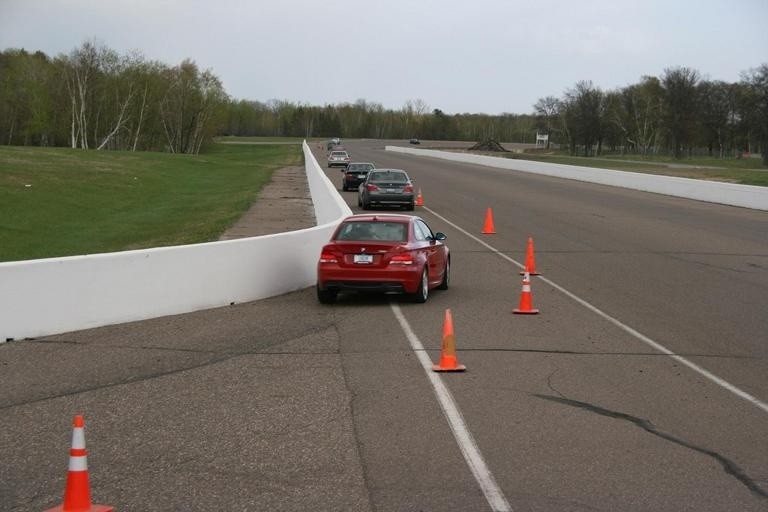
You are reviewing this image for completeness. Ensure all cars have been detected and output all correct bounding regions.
[410,139,421,146]
[326,136,416,211]
[317,212,451,302]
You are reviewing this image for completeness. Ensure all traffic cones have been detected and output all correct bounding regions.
[478,206,497,235]
[51,413,115,512]
[413,185,424,207]
[514,235,542,276]
[509,265,541,315]
[430,306,469,373]
